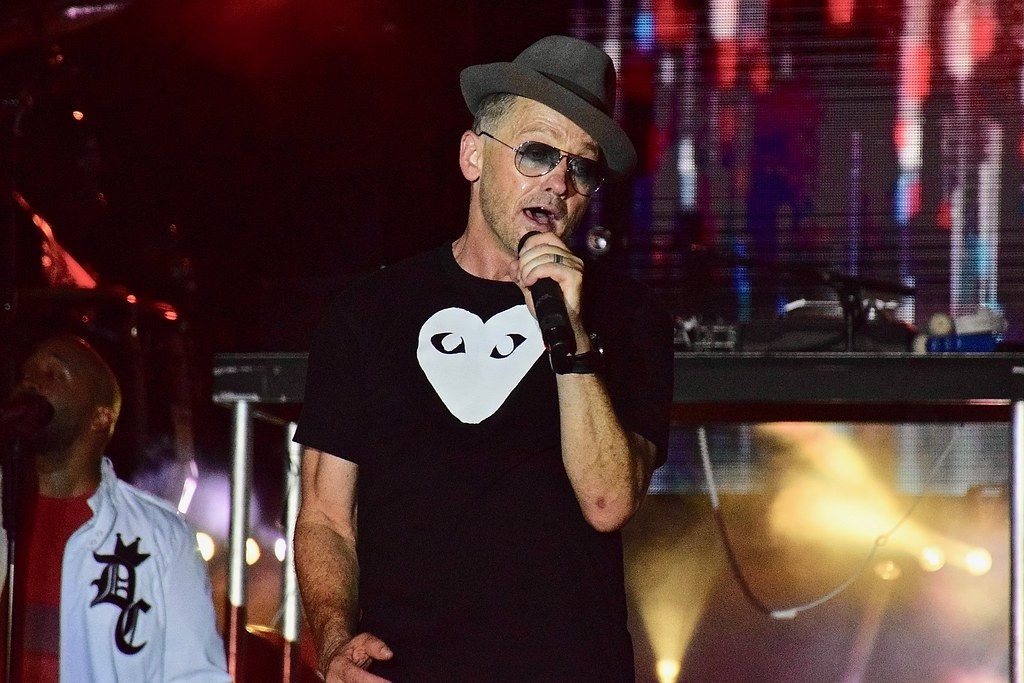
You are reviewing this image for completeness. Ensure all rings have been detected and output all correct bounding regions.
[554,254,564,264]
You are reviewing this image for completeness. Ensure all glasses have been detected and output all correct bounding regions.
[473,131,621,197]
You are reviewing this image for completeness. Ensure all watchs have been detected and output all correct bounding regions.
[548,328,606,374]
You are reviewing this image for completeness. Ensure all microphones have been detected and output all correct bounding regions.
[517,230,577,374]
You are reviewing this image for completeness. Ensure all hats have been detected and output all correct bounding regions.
[459,34,638,182]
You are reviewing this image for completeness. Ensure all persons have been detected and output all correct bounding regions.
[1,334,233,683]
[291,34,674,683]
